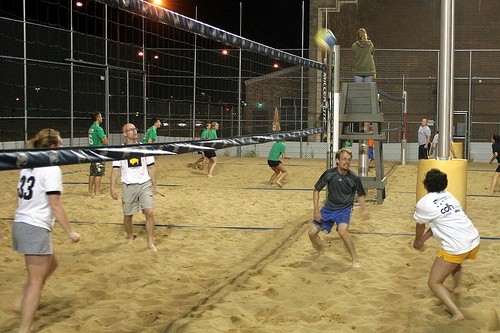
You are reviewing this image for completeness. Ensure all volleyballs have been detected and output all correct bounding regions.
[315,28,337,51]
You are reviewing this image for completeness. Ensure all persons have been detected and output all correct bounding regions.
[143,119,163,161]
[309,149,368,268]
[267,136,291,188]
[412,169,480,322]
[417,118,457,159]
[489,135,500,196]
[110,123,165,253]
[194,121,219,177]
[11,128,80,333]
[88,112,108,195]
[345,122,375,166]
[351,28,376,82]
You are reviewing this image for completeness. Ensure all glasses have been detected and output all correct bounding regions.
[127,127,138,132]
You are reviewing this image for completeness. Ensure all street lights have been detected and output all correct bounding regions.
[138,50,159,97]
